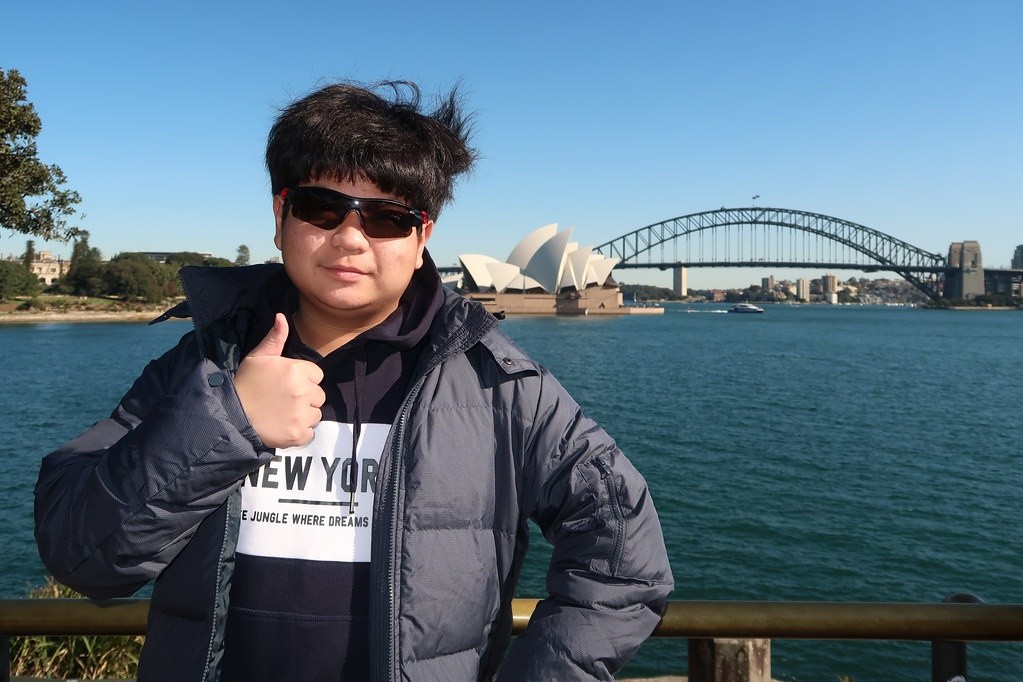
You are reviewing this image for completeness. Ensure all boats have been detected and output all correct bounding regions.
[727,301,764,313]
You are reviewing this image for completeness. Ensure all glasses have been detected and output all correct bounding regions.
[281,186,427,238]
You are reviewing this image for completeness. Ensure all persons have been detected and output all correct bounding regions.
[33,80,674,682]
